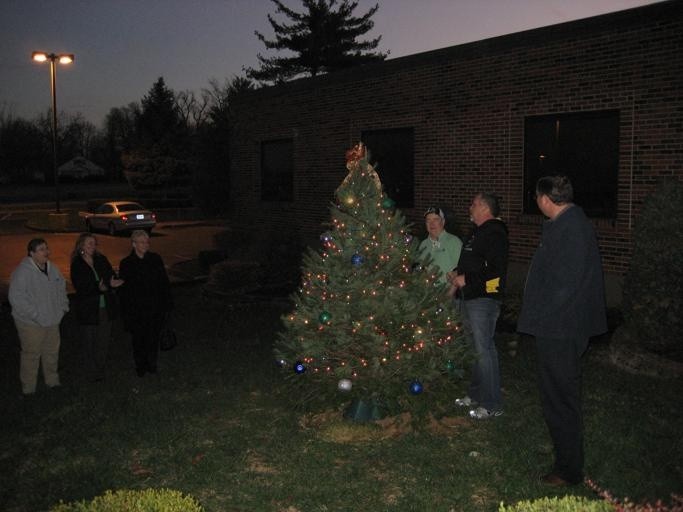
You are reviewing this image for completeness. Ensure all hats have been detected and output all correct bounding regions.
[423,206,446,227]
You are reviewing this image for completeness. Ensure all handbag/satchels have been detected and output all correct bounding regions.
[151,330,178,352]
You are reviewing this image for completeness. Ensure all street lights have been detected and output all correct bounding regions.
[32,51,74,212]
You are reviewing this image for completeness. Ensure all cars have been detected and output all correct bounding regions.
[86,201,156,236]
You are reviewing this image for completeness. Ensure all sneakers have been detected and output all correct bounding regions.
[537,466,584,488]
[454,394,480,409]
[469,405,505,421]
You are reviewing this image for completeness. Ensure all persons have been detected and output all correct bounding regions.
[6,238,71,400]
[514,172,608,488]
[416,206,462,297]
[116,228,172,378]
[444,193,510,421]
[69,232,123,364]
